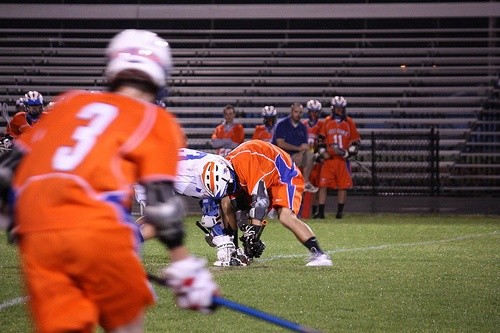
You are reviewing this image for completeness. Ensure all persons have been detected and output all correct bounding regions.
[199,137,336,268]
[209,104,245,157]
[309,94,363,220]
[0,90,45,149]
[133,147,270,268]
[11,28,222,333]
[272,101,310,157]
[304,97,330,218]
[253,105,280,144]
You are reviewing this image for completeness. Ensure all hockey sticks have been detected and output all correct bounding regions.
[146,274,321,333]
[196,221,250,267]
[247,220,267,266]
[339,147,378,180]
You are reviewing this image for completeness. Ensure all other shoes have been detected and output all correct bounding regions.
[336,211,342,218]
[302,180,320,192]
[313,213,325,218]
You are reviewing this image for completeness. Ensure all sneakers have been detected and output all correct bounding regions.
[305,252,333,267]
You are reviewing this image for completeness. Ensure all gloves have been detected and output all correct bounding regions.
[164,258,220,314]
[212,233,238,266]
[240,223,267,258]
[2,137,13,153]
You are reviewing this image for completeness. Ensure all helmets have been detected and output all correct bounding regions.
[201,158,238,199]
[306,99,321,121]
[331,95,347,117]
[104,29,173,100]
[23,90,43,121]
[16,97,24,109]
[261,105,277,128]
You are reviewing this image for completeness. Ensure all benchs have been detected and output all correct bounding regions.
[0,1,500,198]
